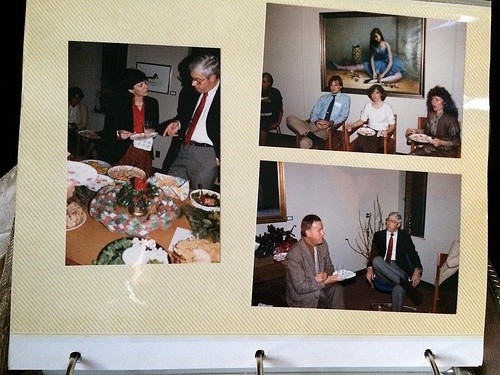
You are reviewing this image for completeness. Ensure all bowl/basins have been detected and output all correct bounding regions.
[190,189,220,212]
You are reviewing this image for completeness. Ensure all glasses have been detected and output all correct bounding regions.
[190,73,215,85]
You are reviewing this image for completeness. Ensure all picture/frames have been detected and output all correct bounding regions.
[256,159,287,224]
[135,61,172,95]
[319,11,426,100]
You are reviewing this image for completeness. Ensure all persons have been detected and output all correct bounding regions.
[110,69,158,179]
[78,80,126,164]
[365,212,423,312]
[438,240,460,314]
[68,86,89,160]
[330,27,408,83]
[346,84,396,153]
[286,75,351,149]
[405,85,461,158]
[163,54,221,189]
[259,72,283,146]
[286,215,345,310]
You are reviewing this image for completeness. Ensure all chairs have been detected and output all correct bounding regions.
[432,252,451,313]
[296,119,345,151]
[346,114,397,154]
[370,277,417,311]
[411,117,461,158]
[267,110,284,144]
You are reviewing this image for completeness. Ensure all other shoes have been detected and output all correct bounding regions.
[310,138,326,148]
[409,293,425,305]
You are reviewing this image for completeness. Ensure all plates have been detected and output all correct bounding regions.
[108,165,145,183]
[67,161,98,186]
[333,270,356,279]
[357,128,375,136]
[66,211,87,231]
[80,159,110,174]
[410,134,429,143]
[87,174,114,192]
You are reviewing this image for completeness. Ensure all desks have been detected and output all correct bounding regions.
[252,252,286,307]
[67,153,195,265]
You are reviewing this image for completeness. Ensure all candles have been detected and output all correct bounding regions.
[134,176,147,189]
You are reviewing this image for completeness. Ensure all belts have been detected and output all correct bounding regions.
[190,140,213,147]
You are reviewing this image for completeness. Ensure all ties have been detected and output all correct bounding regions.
[184,92,208,145]
[385,233,394,264]
[325,95,337,121]
[313,246,320,274]
